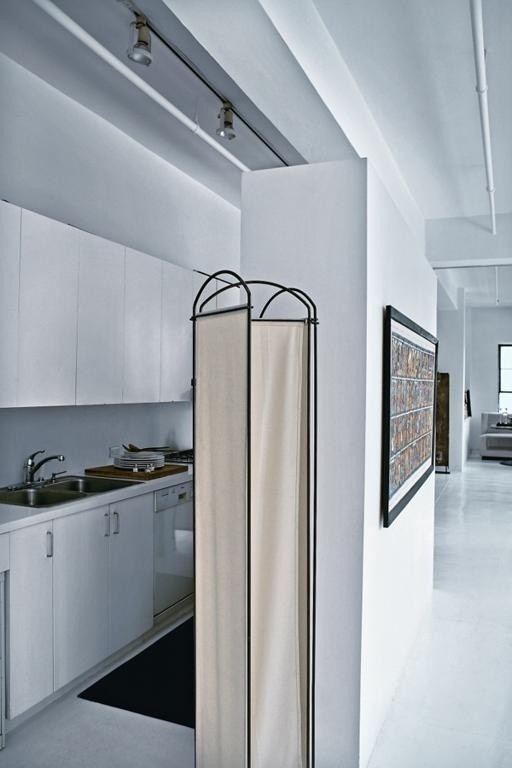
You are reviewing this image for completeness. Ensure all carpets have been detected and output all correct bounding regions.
[78,615,196,729]
[499,461,512,466]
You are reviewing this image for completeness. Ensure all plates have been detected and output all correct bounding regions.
[112,452,166,470]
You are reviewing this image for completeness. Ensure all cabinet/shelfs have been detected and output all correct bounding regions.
[0,481,195,745]
[2,200,239,408]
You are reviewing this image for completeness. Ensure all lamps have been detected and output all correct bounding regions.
[119,0,288,167]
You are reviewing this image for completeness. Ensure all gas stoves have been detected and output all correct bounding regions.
[165,448,193,464]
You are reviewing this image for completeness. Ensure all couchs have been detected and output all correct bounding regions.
[479,412,512,460]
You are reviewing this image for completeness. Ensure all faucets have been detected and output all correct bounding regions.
[24,448,65,487]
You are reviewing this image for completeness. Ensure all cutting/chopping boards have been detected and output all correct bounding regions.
[85,464,188,481]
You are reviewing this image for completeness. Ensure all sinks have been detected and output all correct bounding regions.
[41,476,143,494]
[0,488,78,511]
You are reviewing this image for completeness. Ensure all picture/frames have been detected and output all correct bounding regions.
[383,306,439,528]
